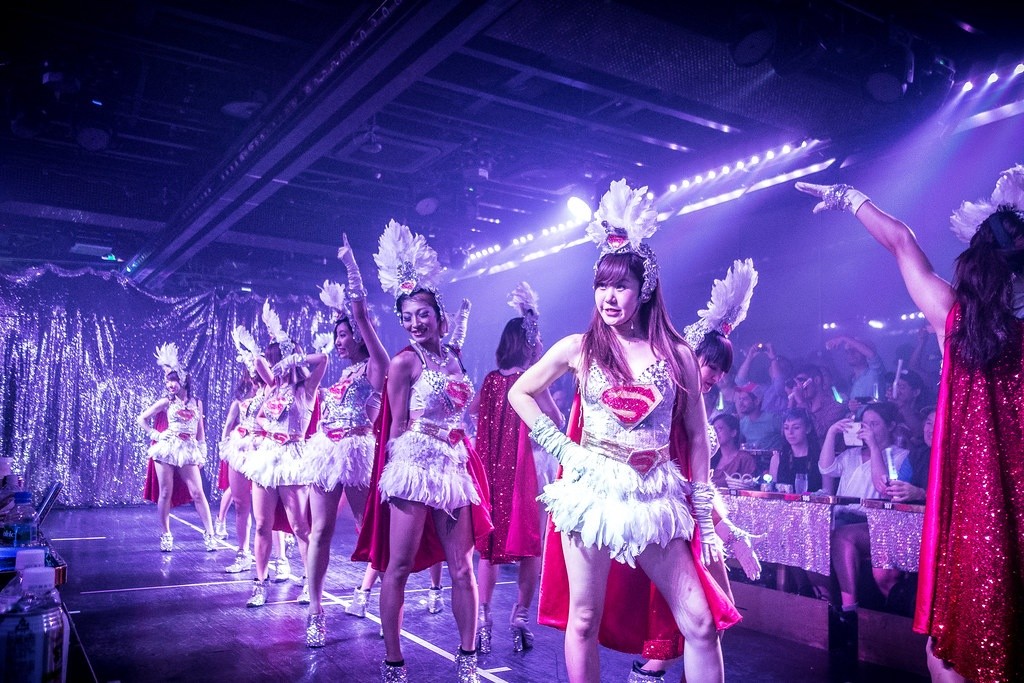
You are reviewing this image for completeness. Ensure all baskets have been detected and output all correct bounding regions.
[726,473,753,489]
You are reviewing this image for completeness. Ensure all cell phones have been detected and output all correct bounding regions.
[786,377,804,389]
[855,396,873,405]
[843,423,862,434]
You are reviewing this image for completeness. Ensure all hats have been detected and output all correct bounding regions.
[735,381,764,403]
[885,369,923,389]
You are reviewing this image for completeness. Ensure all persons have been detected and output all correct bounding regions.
[507,242,725,683]
[350,288,495,683]
[213,233,472,648]
[474,316,566,654]
[795,182,1024,683]
[137,371,218,552]
[461,326,941,644]
[628,330,767,683]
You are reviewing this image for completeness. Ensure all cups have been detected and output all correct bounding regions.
[739,443,759,450]
[795,474,808,494]
[760,483,793,493]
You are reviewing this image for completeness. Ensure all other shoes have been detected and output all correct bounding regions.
[626,660,666,683]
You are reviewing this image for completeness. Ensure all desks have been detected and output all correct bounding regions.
[717,489,928,670]
[0,529,97,683]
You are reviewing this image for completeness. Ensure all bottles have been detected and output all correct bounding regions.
[0,456,40,550]
[0,548,60,612]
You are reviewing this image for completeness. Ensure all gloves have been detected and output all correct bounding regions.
[690,481,724,566]
[199,440,207,469]
[795,182,871,215]
[714,518,767,581]
[337,233,368,301]
[271,354,310,378]
[528,414,592,482]
[150,429,172,441]
[448,298,471,348]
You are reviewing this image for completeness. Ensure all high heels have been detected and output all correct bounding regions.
[455,645,480,683]
[428,584,444,613]
[305,606,327,646]
[246,574,271,606]
[510,602,534,651]
[160,514,252,573]
[275,557,291,581]
[344,585,371,617]
[297,577,310,604]
[475,602,494,653]
[284,534,295,558]
[380,658,408,683]
